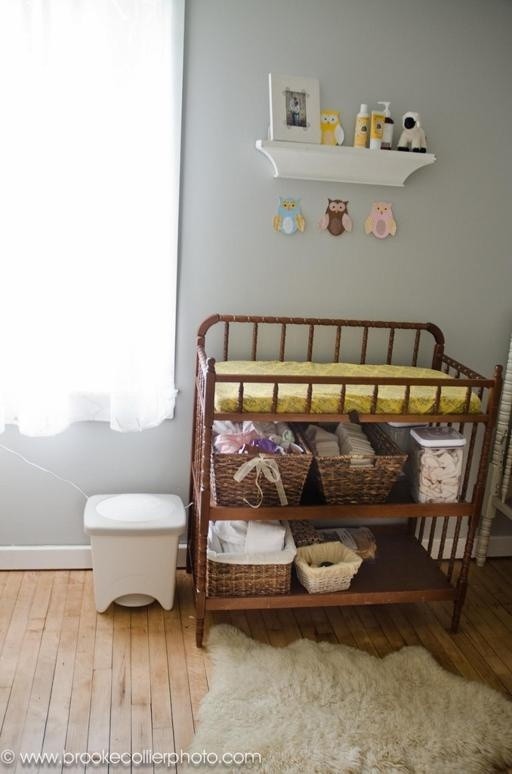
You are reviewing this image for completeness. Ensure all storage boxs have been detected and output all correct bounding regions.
[380,420,430,457]
[407,422,468,507]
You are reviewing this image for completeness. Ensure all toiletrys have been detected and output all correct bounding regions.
[370,109,386,148]
[377,100,395,149]
[354,103,370,149]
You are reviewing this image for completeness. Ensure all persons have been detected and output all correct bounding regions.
[289,96,298,124]
[293,101,300,125]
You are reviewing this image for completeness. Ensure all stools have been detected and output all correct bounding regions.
[83,493,188,614]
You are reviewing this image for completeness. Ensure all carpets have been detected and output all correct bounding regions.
[167,615,512,772]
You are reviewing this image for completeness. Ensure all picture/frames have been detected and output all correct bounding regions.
[267,71,322,146]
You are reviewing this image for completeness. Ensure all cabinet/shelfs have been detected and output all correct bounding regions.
[186,304,505,650]
